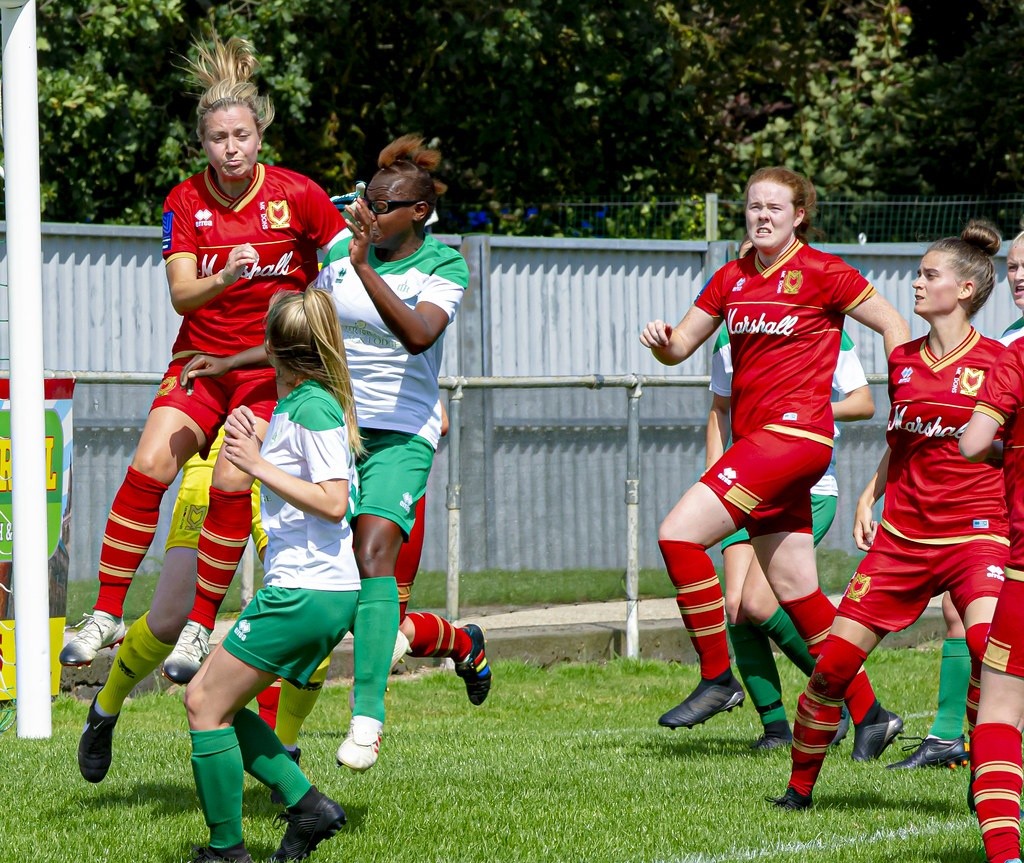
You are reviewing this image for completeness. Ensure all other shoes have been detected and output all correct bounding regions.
[968,777,1024,820]
[983,858,1023,863]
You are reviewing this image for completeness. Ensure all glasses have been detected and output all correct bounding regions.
[363,182,427,214]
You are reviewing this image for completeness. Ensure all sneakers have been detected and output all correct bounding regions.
[336,716,384,775]
[852,700,902,762]
[271,785,348,863]
[830,703,851,746]
[391,630,410,673]
[658,667,746,730]
[161,621,210,686]
[765,787,814,812]
[188,840,253,863]
[454,623,493,706]
[271,748,303,804]
[78,686,121,783]
[59,613,126,669]
[885,735,971,770]
[750,731,792,752]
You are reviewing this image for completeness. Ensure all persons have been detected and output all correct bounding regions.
[184,289,345,863]
[60,30,353,668]
[764,220,1007,812]
[701,230,1024,863]
[638,167,911,762]
[317,131,471,776]
[78,397,492,783]
